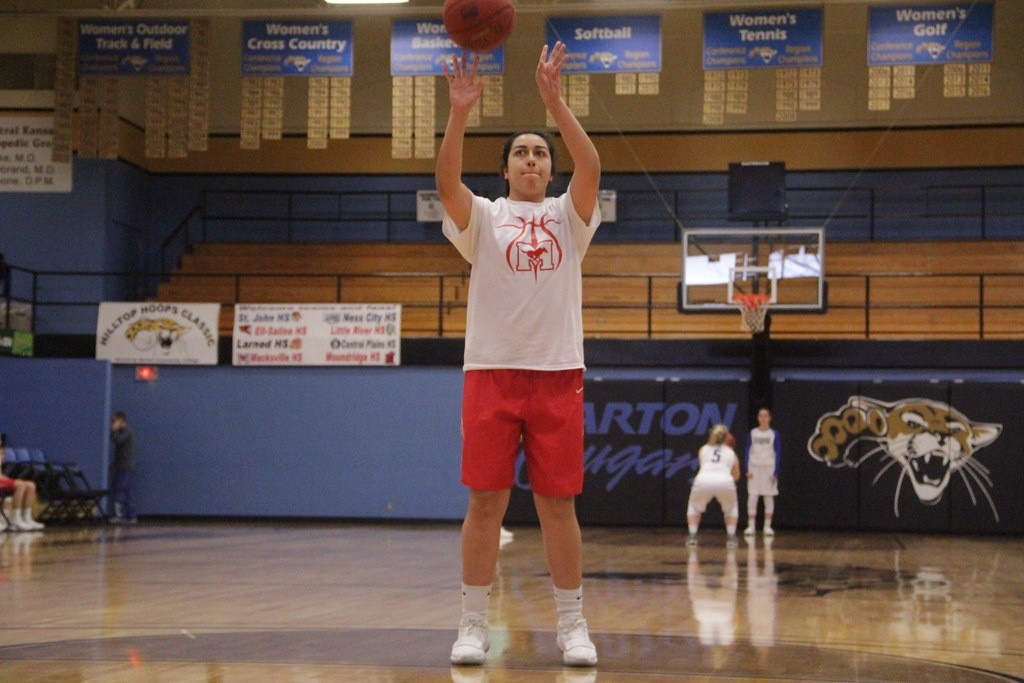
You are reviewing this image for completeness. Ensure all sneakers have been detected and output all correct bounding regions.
[556,612,597,666]
[450,613,490,663]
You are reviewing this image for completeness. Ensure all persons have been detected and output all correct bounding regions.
[686,423,740,545]
[435,39,603,664]
[108,411,136,525]
[685,539,777,671]
[744,408,782,536]
[0,434,44,532]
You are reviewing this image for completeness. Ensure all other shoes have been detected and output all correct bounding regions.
[25,518,45,530]
[726,534,736,541]
[686,534,698,546]
[743,525,756,535]
[108,517,138,525]
[764,527,775,535]
[9,521,32,531]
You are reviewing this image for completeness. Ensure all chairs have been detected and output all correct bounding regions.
[1,459,111,529]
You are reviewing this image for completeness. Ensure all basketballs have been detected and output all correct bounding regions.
[442,0,516,52]
[726,432,736,448]
